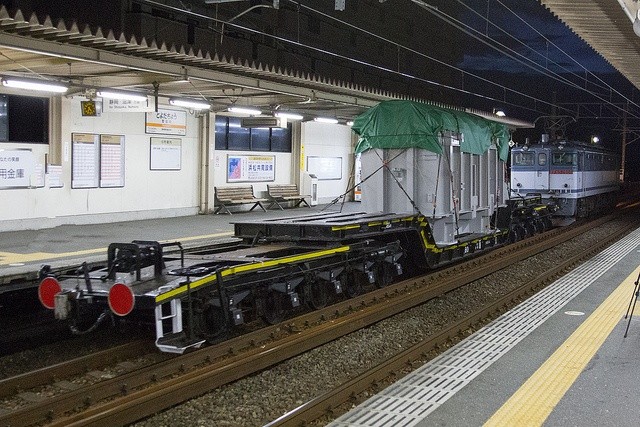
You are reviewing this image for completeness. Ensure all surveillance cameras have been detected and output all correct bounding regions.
[268,102,279,111]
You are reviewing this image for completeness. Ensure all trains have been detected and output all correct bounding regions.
[32,99,620,355]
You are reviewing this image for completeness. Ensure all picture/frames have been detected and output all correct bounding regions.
[149,136,183,171]
[144,107,188,137]
[70,132,101,190]
[306,155,345,181]
[99,133,126,189]
[226,153,277,184]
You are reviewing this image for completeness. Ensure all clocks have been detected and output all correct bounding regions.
[80,99,102,118]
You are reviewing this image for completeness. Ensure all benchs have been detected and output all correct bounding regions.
[265,183,313,212]
[213,184,271,216]
